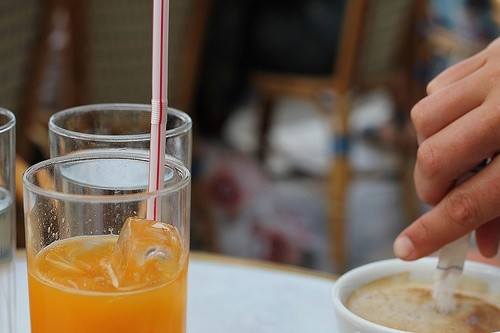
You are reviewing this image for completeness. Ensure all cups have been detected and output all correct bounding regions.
[1,107,17,333]
[22,147,191,333]
[49,103,193,240]
[330,256,500,332]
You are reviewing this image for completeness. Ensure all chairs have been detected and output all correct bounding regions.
[248,0,425,278]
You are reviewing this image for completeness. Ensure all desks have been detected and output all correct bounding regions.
[0,251,338,333]
